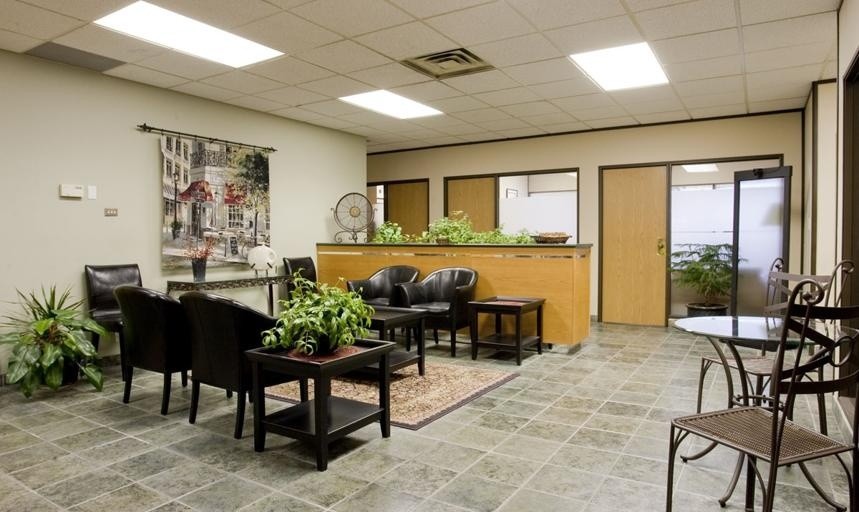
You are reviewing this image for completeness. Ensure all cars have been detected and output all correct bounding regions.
[202,227,234,243]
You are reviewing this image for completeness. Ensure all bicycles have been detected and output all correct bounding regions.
[241,231,256,259]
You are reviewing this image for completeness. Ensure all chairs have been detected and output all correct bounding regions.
[84,262,147,381]
[112,284,188,415]
[177,290,309,440]
[697,258,856,439]
[282,256,319,307]
[347,265,421,342]
[399,265,479,359]
[665,279,859,512]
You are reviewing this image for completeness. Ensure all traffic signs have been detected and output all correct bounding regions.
[191,192,205,202]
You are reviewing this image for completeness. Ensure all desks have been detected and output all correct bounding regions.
[354,306,429,383]
[164,272,295,317]
[671,316,859,507]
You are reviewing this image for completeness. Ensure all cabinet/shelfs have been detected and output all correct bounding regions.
[243,342,398,472]
[468,295,547,366]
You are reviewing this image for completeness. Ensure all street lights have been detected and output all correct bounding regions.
[172,172,179,228]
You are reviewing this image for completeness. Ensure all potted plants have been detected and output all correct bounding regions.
[666,242,748,316]
[178,233,219,283]
[0,281,109,398]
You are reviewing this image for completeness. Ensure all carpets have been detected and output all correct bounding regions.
[188,363,522,432]
